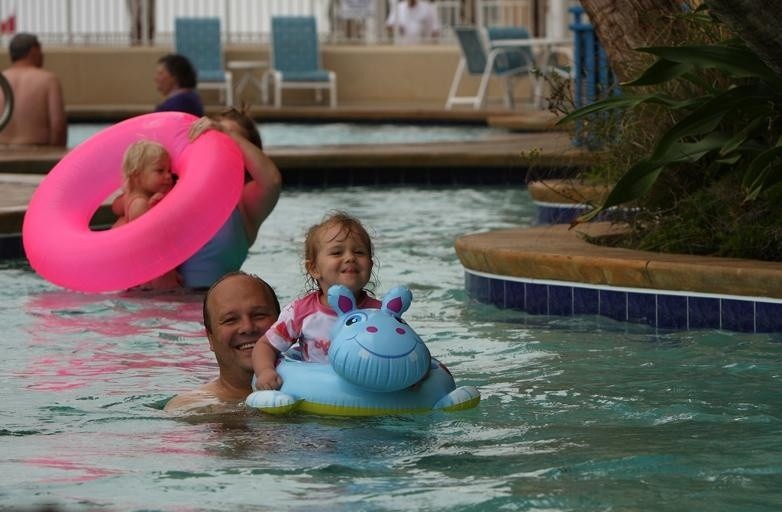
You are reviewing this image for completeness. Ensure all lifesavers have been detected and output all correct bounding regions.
[21,111,245,293]
[244,284,479,416]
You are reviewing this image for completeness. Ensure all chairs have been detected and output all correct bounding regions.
[171,14,338,110]
[442,24,577,114]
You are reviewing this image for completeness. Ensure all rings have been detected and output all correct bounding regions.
[199,125,201,128]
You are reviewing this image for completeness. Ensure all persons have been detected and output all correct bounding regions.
[0,33,66,145]
[154,54,203,117]
[162,271,281,411]
[386,0,442,45]
[252,209,381,390]
[110,106,282,291]
[112,139,173,222]
